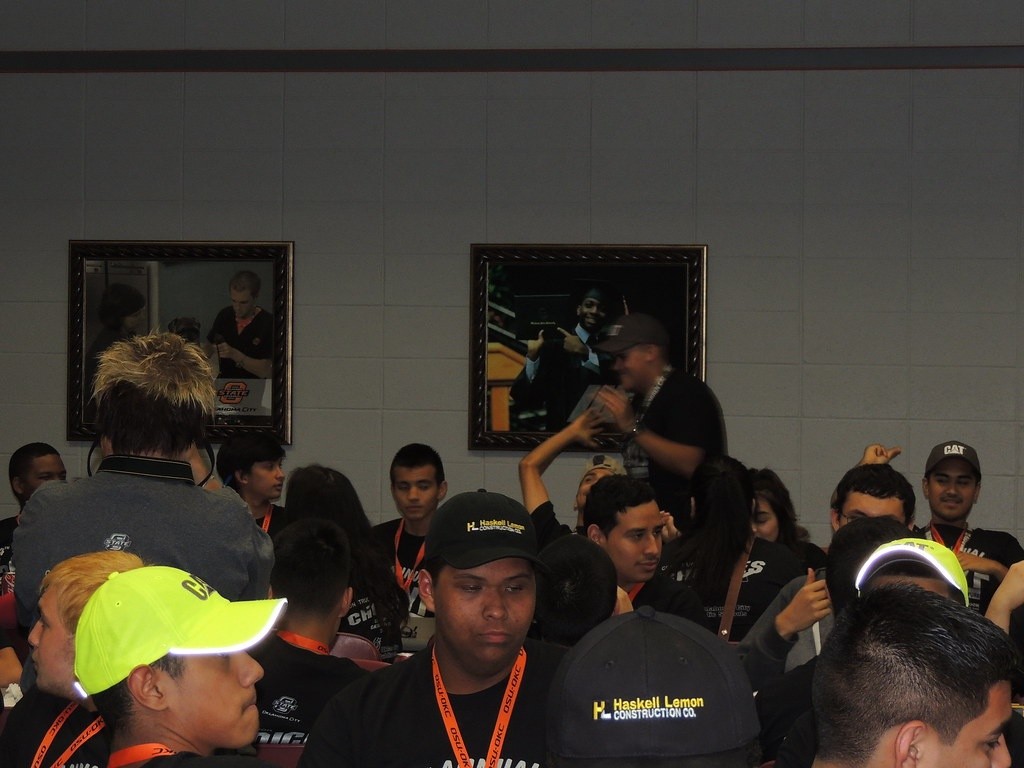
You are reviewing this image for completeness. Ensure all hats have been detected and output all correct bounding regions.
[598,317,661,353]
[557,597,756,761]
[925,440,980,477]
[853,536,970,608]
[426,488,541,571]
[580,454,626,487]
[74,566,288,694]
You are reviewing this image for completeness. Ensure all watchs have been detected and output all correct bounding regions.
[623,420,646,444]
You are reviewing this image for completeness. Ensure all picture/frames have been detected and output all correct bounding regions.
[64,237,297,449]
[467,240,709,453]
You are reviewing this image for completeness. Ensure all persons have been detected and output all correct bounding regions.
[811,579,1018,767]
[0,626,23,689]
[200,270,277,380]
[596,311,730,541]
[545,605,764,768]
[980,559,1023,639]
[253,515,371,768]
[215,431,448,655]
[487,275,619,436]
[82,283,148,430]
[516,408,828,648]
[298,488,585,768]
[15,334,276,633]
[0,550,143,768]
[737,465,917,757]
[857,440,1023,642]
[74,561,280,768]
[1,442,68,664]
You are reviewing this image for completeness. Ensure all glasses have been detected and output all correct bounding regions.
[839,513,859,525]
